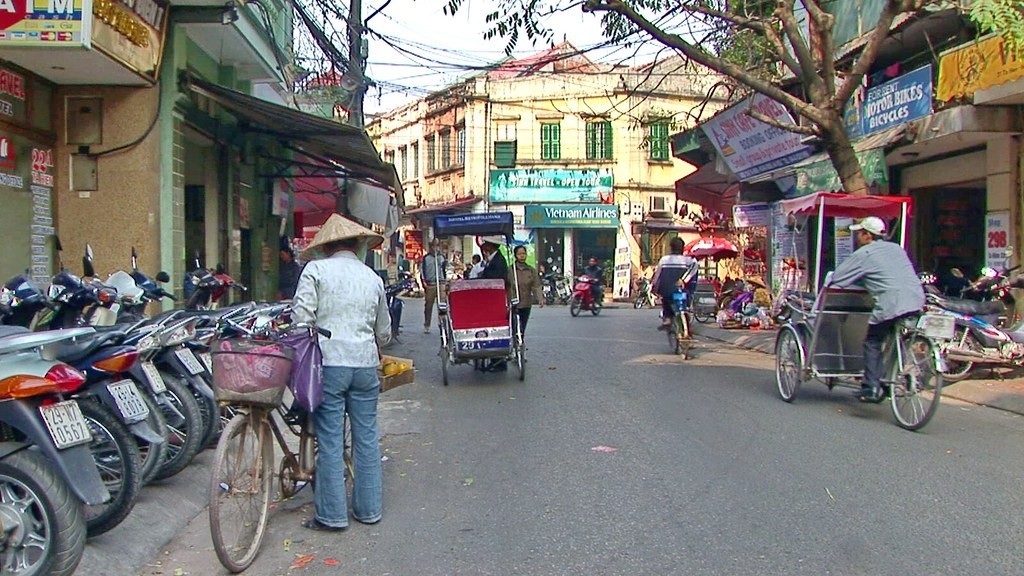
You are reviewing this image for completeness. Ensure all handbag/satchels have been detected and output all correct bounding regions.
[275,327,323,413]
[213,340,288,393]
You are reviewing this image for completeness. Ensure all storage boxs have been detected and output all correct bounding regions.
[378,368,415,392]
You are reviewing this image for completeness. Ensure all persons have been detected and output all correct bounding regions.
[453,241,508,289]
[653,238,699,339]
[508,245,544,364]
[420,236,447,333]
[578,257,603,308]
[825,216,926,403]
[273,247,300,300]
[290,210,392,532]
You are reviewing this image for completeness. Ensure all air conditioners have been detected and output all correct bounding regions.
[649,196,670,213]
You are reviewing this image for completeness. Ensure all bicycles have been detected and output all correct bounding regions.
[208,318,356,573]
[647,277,697,360]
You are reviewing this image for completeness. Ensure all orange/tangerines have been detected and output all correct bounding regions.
[383,357,410,377]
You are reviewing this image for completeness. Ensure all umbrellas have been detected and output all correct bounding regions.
[683,237,739,278]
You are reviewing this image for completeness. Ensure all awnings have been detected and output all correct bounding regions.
[404,196,482,215]
[187,76,405,236]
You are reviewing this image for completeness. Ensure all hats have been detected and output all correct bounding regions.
[301,213,385,260]
[482,235,504,245]
[849,216,886,236]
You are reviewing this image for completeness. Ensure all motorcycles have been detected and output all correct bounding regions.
[0,240,292,576]
[908,245,1024,382]
[570,274,606,317]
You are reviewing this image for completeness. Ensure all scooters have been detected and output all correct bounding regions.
[376,265,419,346]
[539,265,572,306]
[634,272,661,308]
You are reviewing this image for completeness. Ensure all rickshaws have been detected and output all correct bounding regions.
[771,191,946,431]
[432,212,529,386]
[691,283,718,322]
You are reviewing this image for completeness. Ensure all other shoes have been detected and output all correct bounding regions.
[658,322,671,330]
[487,362,508,372]
[594,302,600,308]
[304,518,339,531]
[854,385,878,398]
[425,326,431,333]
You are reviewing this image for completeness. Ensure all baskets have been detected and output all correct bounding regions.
[209,339,298,409]
[378,365,415,393]
